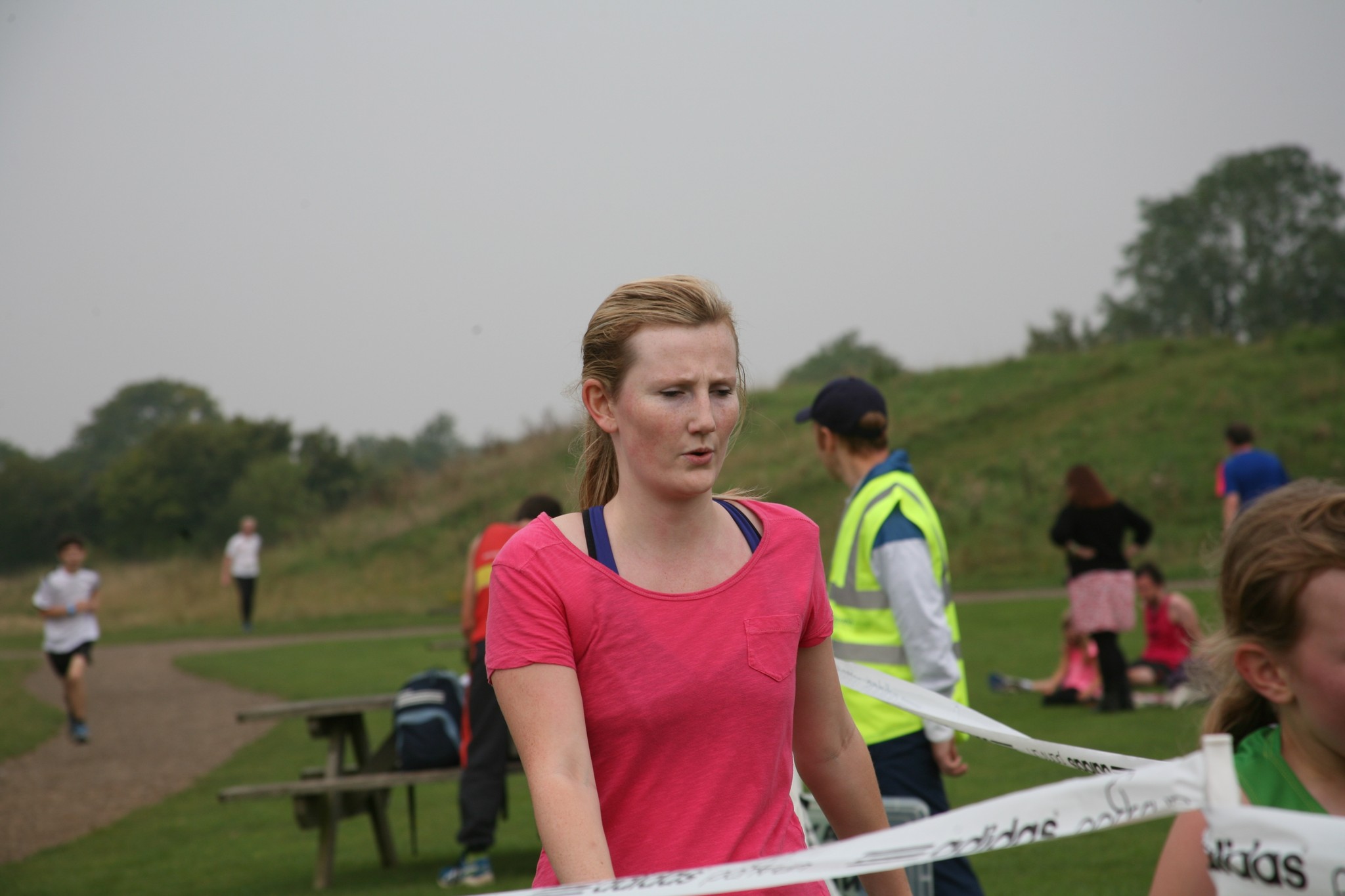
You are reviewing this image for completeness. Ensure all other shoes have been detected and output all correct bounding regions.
[71,724,89,743]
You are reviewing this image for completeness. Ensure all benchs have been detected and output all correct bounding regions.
[216,770,459,890]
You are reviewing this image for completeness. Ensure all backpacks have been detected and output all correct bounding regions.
[388,671,464,772]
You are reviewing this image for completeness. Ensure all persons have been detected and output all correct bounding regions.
[1046,463,1154,712]
[433,495,564,891]
[483,276,916,896]
[1148,476,1345,896]
[1215,421,1289,528]
[31,538,103,745]
[794,377,983,896]
[988,558,1202,694]
[219,516,263,634]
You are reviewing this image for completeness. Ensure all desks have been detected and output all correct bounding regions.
[232,692,396,890]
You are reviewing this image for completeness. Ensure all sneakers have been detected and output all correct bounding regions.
[438,858,494,889]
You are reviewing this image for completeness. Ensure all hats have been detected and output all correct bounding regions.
[794,377,889,439]
